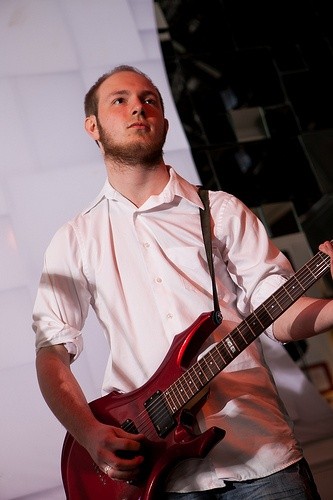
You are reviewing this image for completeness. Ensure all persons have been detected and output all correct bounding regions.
[32,64,333,499]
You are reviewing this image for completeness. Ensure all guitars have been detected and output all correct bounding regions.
[60,238,333,500]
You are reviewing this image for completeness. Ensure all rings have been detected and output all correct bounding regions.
[104,466,112,476]
